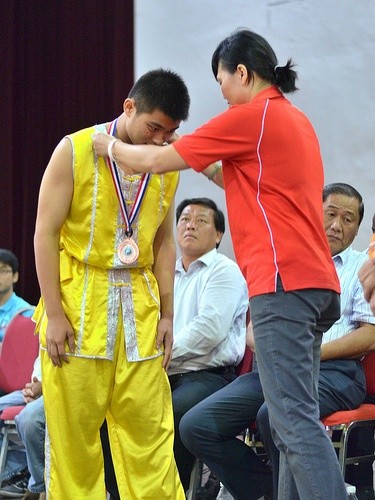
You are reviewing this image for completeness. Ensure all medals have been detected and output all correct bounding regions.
[117,239,139,265]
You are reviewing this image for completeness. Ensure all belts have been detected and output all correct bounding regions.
[168,365,236,383]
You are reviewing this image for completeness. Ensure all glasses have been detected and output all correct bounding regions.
[0,266,12,273]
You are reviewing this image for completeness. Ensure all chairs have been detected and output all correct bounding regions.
[189,306,375,500]
[0,306,40,500]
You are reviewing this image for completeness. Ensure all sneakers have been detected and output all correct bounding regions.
[0,477,29,498]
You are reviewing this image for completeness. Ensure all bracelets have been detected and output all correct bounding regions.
[208,164,220,180]
[107,138,122,162]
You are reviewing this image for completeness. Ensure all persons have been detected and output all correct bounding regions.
[0,250,36,342]
[91,29,350,500]
[0,350,46,496]
[179,183,375,500]
[96,197,251,500]
[33,67,191,499]
